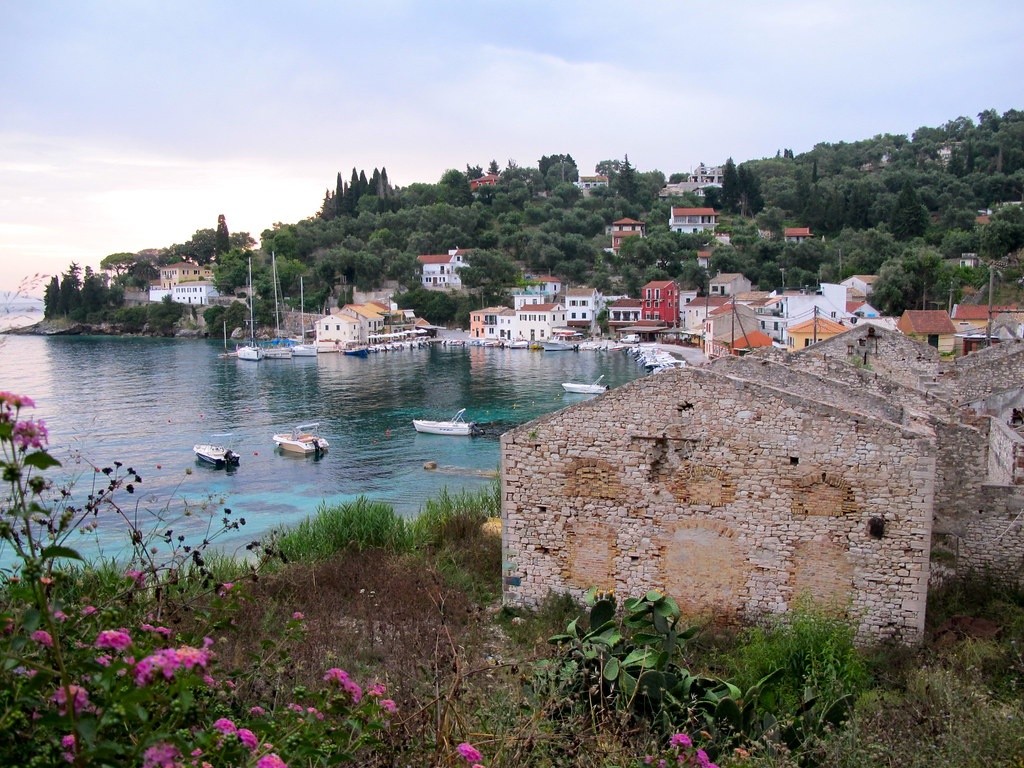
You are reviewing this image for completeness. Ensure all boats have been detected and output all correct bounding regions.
[413,408,474,436]
[273,423,329,453]
[368,328,431,352]
[541,330,584,351]
[338,339,370,357]
[192,432,240,473]
[442,336,545,349]
[561,374,610,394]
[578,337,624,351]
[626,343,687,375]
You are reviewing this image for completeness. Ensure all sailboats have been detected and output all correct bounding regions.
[235,257,264,360]
[292,276,318,357]
[262,251,294,359]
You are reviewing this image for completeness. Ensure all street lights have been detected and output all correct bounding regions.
[780,268,785,297]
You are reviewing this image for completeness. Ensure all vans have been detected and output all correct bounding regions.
[620,334,640,344]
